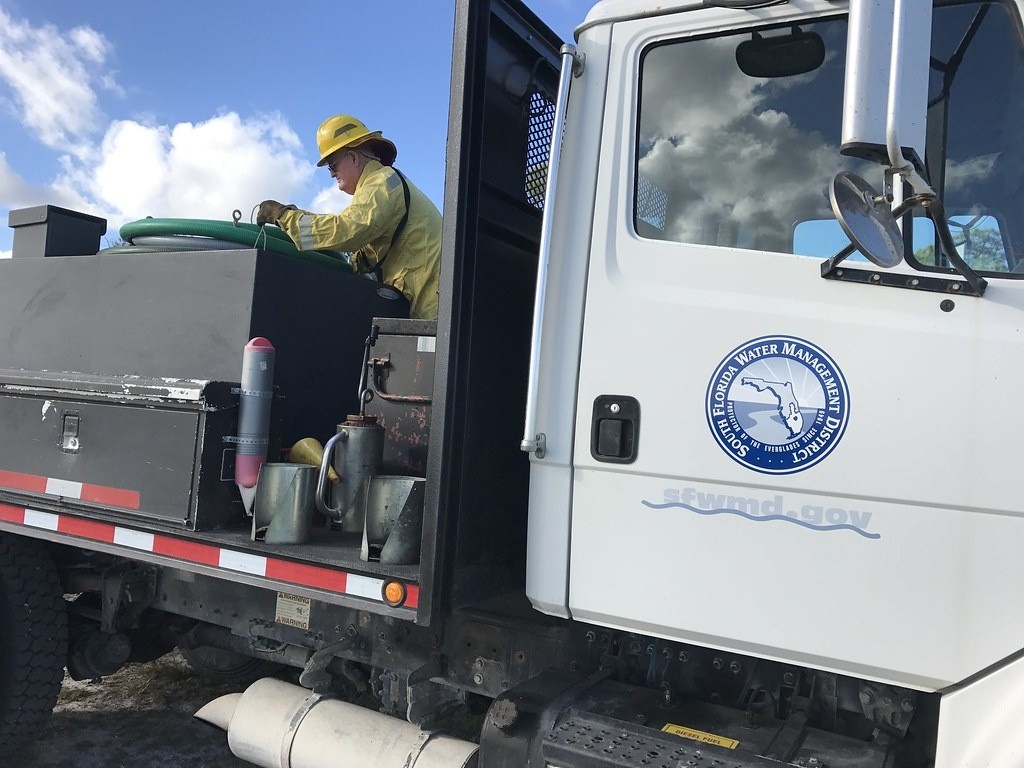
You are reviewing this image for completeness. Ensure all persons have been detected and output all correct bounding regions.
[256,114,441,321]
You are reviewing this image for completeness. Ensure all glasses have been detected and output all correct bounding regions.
[327,153,351,171]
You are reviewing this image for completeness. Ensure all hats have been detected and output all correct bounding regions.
[315,114,398,173]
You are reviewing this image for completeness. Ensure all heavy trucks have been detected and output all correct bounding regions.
[0,2,1024,766]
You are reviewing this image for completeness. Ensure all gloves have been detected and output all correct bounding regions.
[256,200,299,229]
